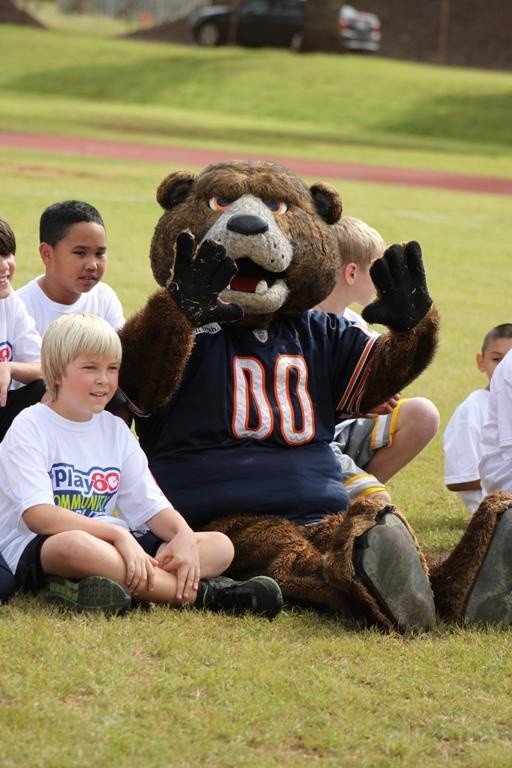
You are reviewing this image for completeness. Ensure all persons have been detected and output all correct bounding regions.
[0,219,48,442]
[440,323,512,515]
[313,213,440,506]
[11,200,126,341]
[477,348,512,498]
[0,312,284,619]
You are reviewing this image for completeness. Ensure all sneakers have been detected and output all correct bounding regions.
[41,573,134,617]
[195,573,285,621]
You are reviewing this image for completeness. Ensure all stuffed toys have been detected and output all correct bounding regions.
[117,158,512,636]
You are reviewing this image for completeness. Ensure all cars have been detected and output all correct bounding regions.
[186,0,384,53]
